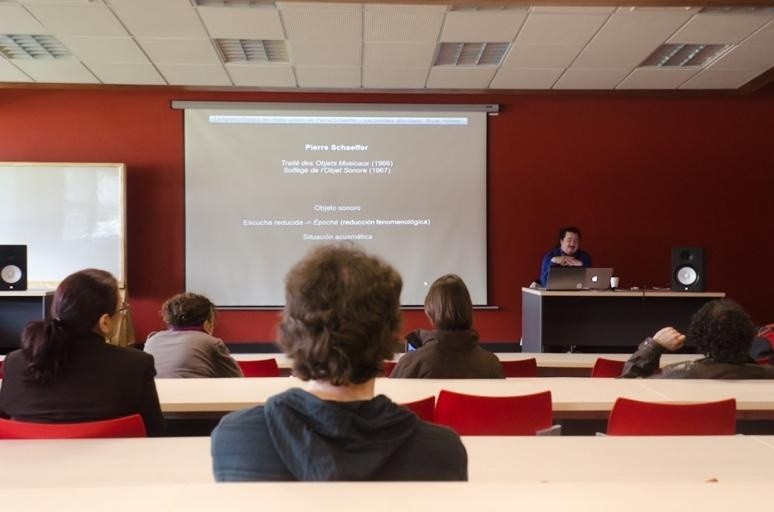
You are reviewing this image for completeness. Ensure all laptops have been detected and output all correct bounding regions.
[583,267,614,291]
[530,265,585,291]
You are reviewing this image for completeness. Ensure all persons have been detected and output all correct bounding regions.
[618,301,773,378]
[539,224,591,289]
[0,266,168,436]
[386,272,508,378]
[206,238,469,481]
[143,292,244,377]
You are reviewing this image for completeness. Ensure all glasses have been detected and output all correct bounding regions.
[109,301,130,317]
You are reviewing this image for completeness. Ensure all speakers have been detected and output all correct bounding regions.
[671,247,705,293]
[0,244,28,291]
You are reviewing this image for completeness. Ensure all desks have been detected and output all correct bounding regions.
[0,288,57,356]
[522,284,725,355]
